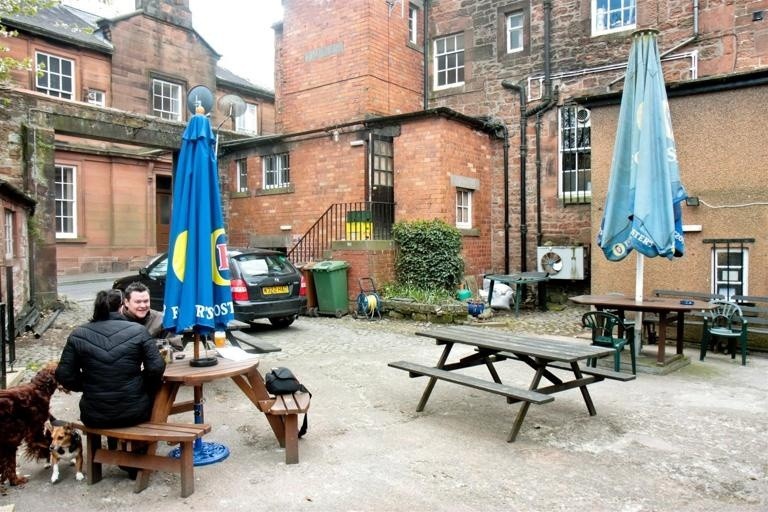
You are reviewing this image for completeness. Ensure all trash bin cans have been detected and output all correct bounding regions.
[295,261,350,317]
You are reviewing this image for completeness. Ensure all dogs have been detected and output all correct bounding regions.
[42,413,86,485]
[0,360,73,496]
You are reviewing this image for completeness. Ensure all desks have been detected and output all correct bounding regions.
[567,294,720,363]
[133,349,289,494]
[176,319,252,351]
[413,327,617,443]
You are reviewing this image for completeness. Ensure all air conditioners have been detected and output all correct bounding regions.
[536,246,584,280]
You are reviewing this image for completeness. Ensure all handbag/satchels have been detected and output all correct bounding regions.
[265,367,299,395]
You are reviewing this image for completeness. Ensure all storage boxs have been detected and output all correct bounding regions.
[345,211,373,241]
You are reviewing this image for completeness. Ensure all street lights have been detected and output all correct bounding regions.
[186,84,247,167]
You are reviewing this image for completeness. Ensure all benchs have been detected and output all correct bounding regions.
[387,360,555,404]
[231,331,283,354]
[727,295,768,355]
[71,417,211,498]
[643,289,725,354]
[257,392,311,465]
[473,347,636,382]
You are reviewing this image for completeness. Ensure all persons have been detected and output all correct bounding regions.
[115,281,184,360]
[53,289,167,481]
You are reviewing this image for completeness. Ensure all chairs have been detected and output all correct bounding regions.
[581,311,636,376]
[605,291,635,351]
[699,300,748,366]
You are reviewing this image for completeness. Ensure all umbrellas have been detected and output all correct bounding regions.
[162,113,236,449]
[595,27,690,357]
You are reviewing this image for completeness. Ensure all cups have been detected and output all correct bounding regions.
[214,331,226,349]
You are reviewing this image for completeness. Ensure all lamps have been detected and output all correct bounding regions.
[686,197,699,206]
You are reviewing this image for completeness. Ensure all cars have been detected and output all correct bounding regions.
[112,245,306,328]
